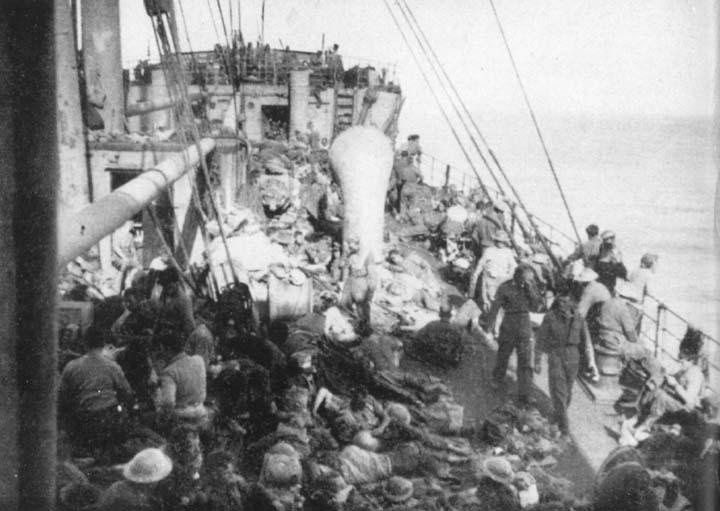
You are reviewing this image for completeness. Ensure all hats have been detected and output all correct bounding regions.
[493,230,511,243]
[482,457,515,485]
[122,448,172,486]
[383,473,413,504]
